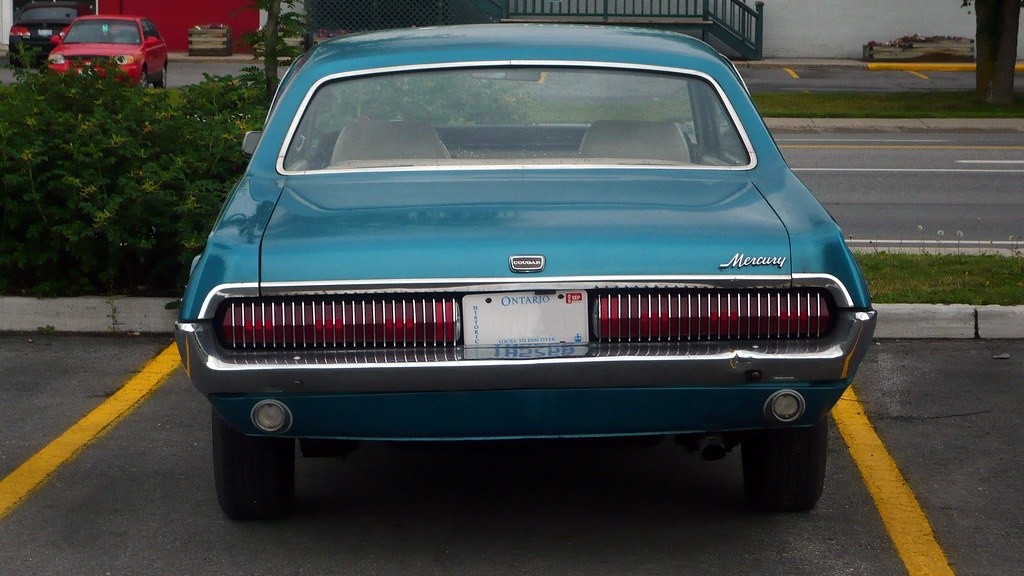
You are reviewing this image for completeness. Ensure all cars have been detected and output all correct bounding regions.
[48,14,169,91]
[175,21,879,525]
[8,4,82,70]
